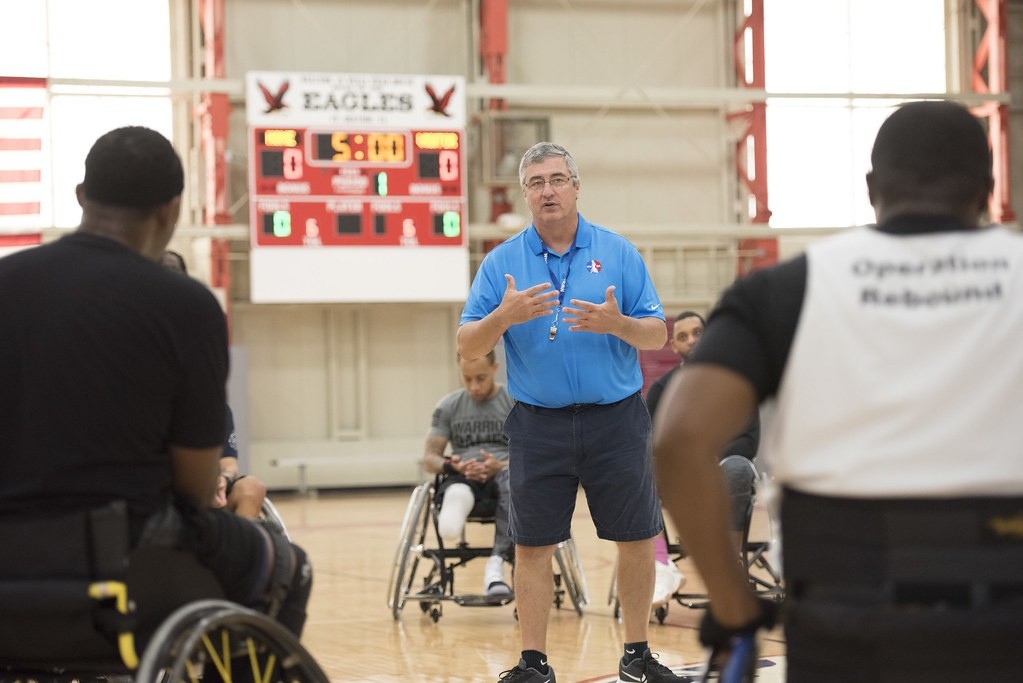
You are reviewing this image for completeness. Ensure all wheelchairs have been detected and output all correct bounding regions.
[387,469,589,623]
[698,496,1023,682]
[1,494,331,683]
[605,455,784,625]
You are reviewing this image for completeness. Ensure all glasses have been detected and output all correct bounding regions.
[524,176,576,190]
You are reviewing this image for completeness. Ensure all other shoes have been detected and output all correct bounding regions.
[485,581,512,597]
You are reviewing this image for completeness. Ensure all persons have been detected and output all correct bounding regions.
[458,143,692,683]
[0,126,313,683]
[423,350,516,603]
[653,102,1022,683]
[647,312,760,609]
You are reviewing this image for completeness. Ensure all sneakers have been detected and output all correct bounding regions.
[651,559,686,608]
[617,648,694,683]
[496,658,556,683]
